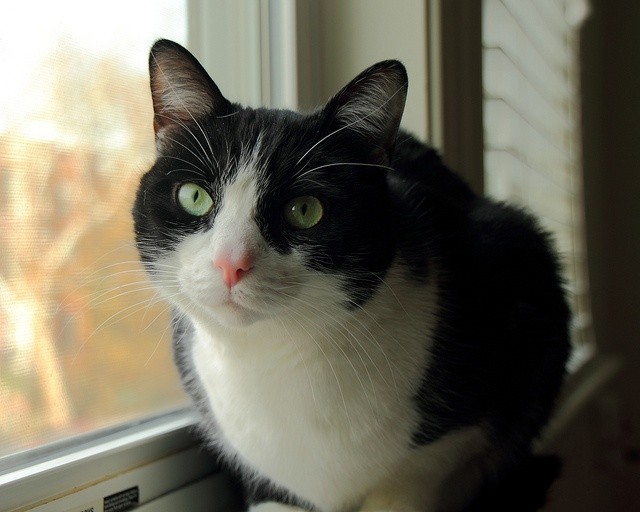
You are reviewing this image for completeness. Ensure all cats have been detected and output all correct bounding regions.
[50,39,574,512]
